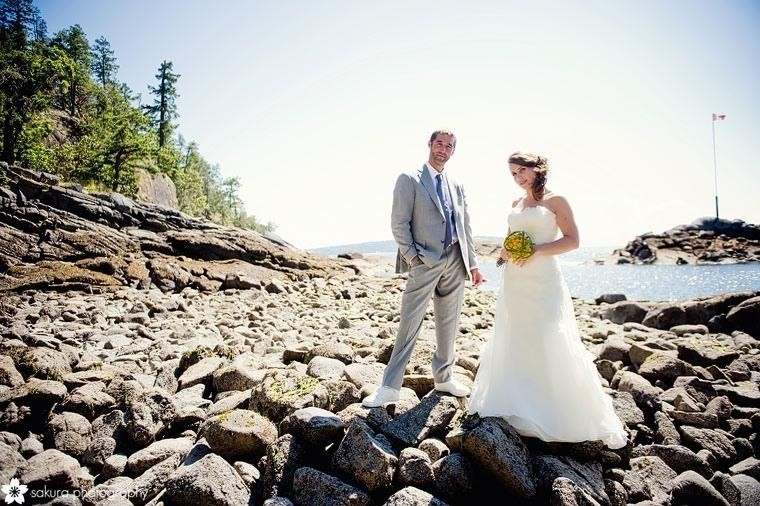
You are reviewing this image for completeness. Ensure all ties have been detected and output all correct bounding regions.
[434,173,452,248]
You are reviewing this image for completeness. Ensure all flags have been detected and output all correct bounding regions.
[712,113,726,121]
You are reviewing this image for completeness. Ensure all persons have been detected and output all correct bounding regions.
[464,150,630,449]
[361,128,483,407]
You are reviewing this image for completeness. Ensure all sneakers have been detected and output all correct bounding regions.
[432,378,471,398]
[361,386,400,410]
